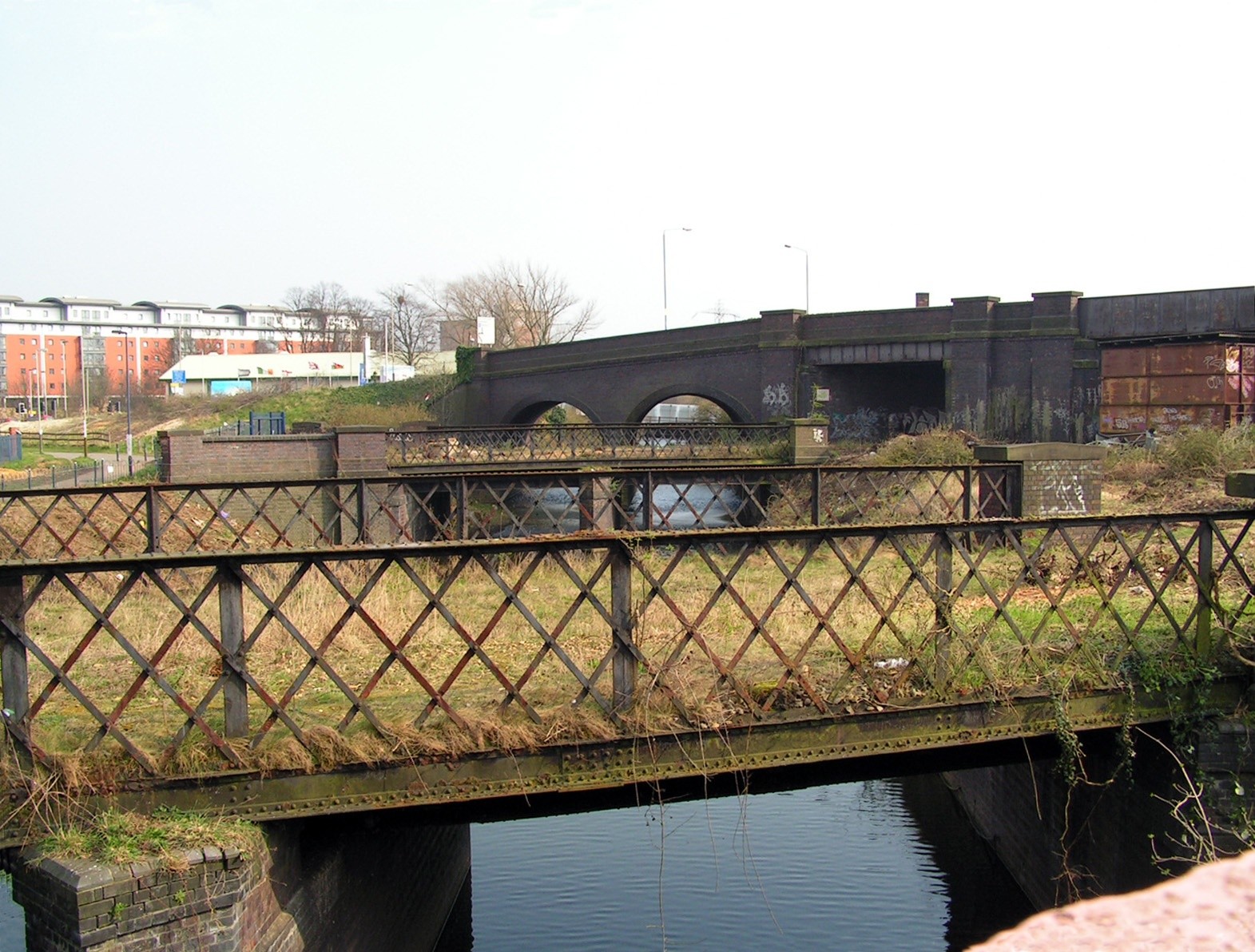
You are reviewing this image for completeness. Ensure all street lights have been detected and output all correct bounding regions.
[663,227,693,330]
[784,244,809,315]
[112,330,134,479]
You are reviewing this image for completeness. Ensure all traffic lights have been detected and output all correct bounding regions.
[19,403,25,414]
[107,401,113,412]
[114,401,121,412]
